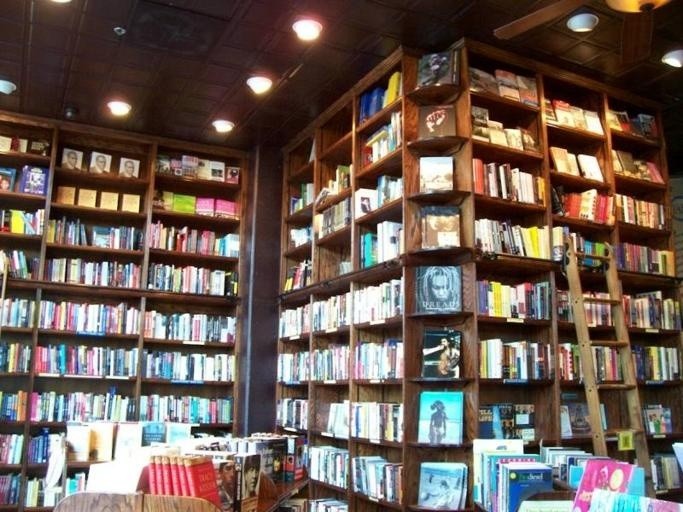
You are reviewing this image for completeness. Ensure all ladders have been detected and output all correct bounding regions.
[561,236,657,502]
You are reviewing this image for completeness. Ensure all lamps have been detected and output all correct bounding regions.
[291,20,323,41]
[567,14,599,32]
[246,76,272,94]
[107,101,131,116]
[0,80,17,95]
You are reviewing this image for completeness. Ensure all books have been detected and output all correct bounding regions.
[417,392,468,510]
[418,51,459,138]
[0,135,237,511]
[472,67,683,512]
[277,72,402,510]
[416,265,462,377]
[420,157,461,248]
[230,430,305,484]
